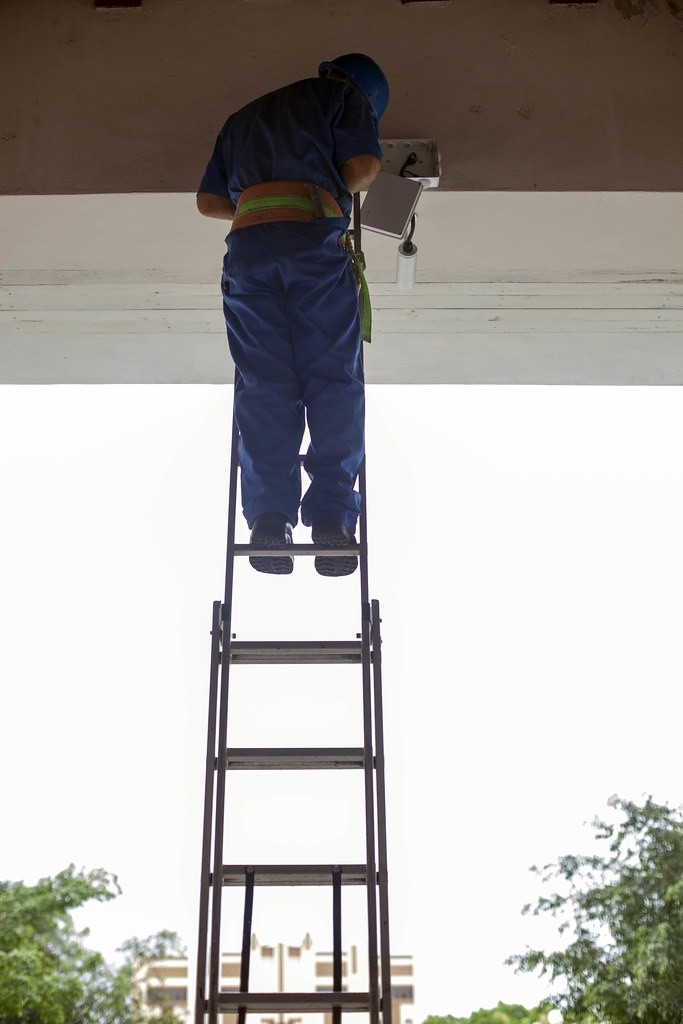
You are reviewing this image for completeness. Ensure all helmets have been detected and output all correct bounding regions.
[318,52,390,140]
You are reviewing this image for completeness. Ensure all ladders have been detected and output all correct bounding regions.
[196,192,392,1024]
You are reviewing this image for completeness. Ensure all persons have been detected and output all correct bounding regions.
[196,53,390,575]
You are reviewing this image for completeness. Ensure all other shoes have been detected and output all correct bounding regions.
[249,513,293,574]
[312,518,359,577]
